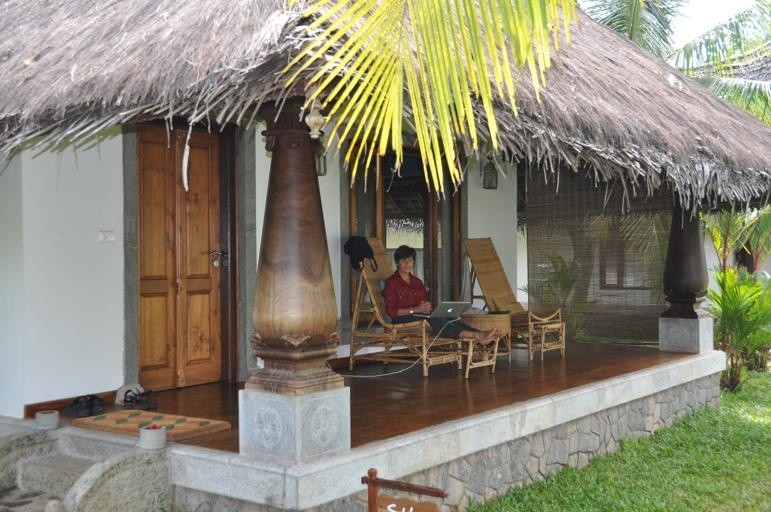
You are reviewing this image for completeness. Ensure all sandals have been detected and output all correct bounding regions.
[123,390,156,410]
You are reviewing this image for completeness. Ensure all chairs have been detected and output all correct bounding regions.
[347,236,566,379]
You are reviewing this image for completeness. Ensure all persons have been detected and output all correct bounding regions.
[380,244,504,345]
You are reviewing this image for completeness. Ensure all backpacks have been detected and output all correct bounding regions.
[344,235,378,272]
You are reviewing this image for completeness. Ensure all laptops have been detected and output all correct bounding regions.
[413,302,471,319]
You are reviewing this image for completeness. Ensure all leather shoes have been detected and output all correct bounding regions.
[64,394,104,417]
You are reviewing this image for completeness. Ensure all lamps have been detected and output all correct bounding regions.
[313,139,328,177]
[483,156,499,189]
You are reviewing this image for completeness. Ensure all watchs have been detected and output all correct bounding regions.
[409,305,413,314]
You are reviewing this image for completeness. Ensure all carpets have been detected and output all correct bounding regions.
[71,408,232,442]
